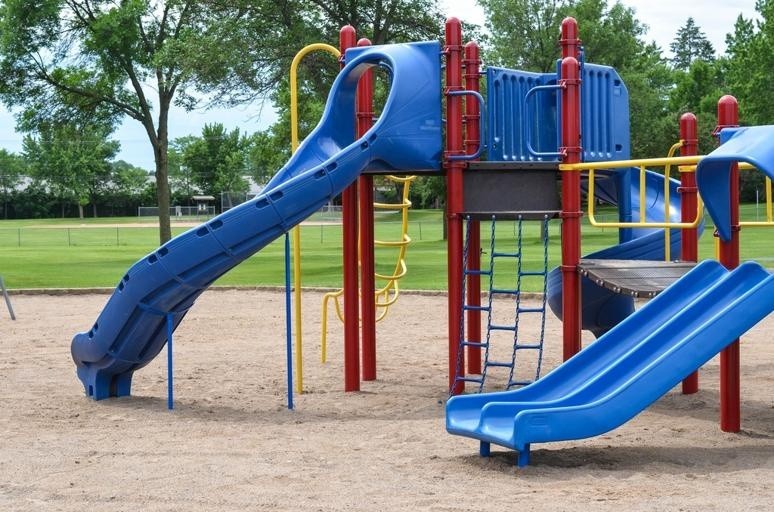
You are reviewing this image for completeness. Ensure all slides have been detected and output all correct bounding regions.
[545,167,706,338]
[446,258,774,468]
[70,41,440,400]
